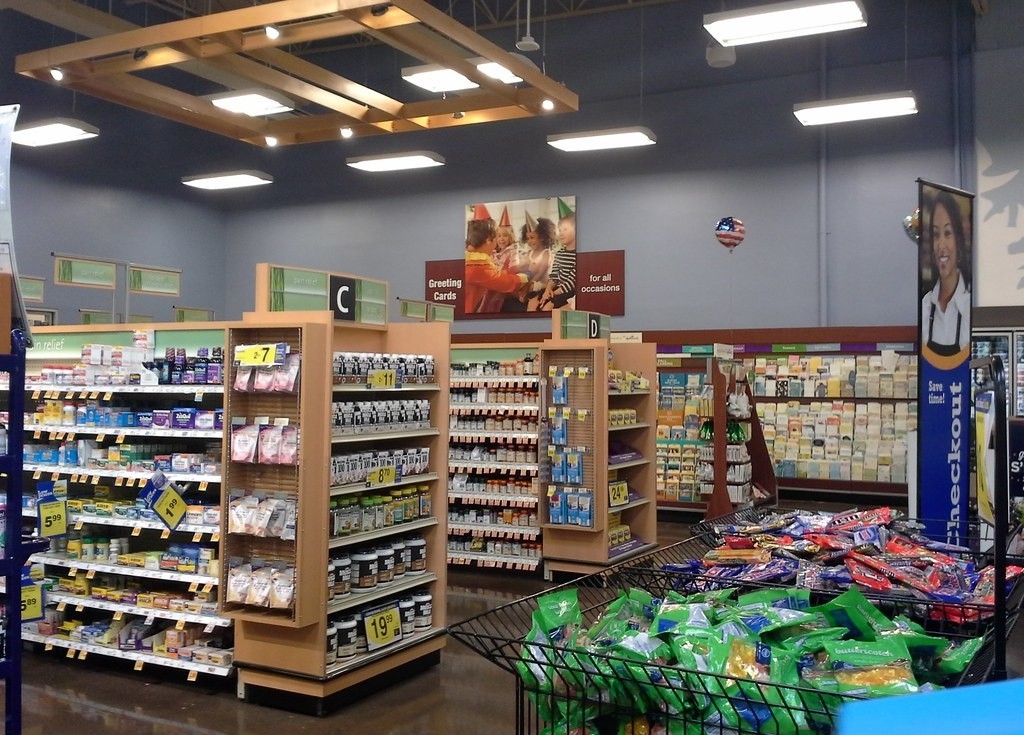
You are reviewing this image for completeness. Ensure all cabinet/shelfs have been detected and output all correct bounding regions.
[775,350,921,508]
[217,324,457,719]
[542,335,659,589]
[444,342,550,580]
[0,321,242,697]
[657,356,780,530]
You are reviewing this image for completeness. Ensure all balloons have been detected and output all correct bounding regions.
[715,216,746,248]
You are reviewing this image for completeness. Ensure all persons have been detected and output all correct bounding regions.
[920,187,970,369]
[465,212,576,312]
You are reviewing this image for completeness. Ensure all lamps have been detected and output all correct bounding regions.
[263,23,282,41]
[702,0,871,48]
[180,170,275,192]
[791,89,921,127]
[452,110,465,119]
[199,87,297,118]
[12,117,101,148]
[546,126,658,153]
[48,66,64,82]
[401,52,540,93]
[346,150,446,172]
[132,47,149,62]
[372,4,389,18]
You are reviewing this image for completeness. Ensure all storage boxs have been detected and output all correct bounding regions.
[661,370,704,395]
[696,443,753,504]
[547,376,594,529]
[624,368,650,392]
[607,478,630,508]
